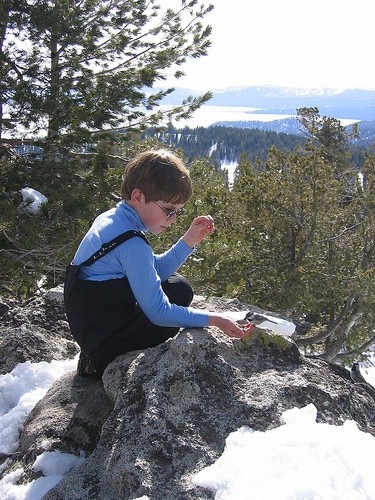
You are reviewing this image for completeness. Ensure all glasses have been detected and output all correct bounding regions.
[151,199,185,218]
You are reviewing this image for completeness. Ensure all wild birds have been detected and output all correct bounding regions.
[244,312,278,325]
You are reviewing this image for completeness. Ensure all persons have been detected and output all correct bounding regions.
[63,149,256,380]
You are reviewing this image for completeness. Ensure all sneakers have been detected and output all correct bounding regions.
[77,347,98,376]
